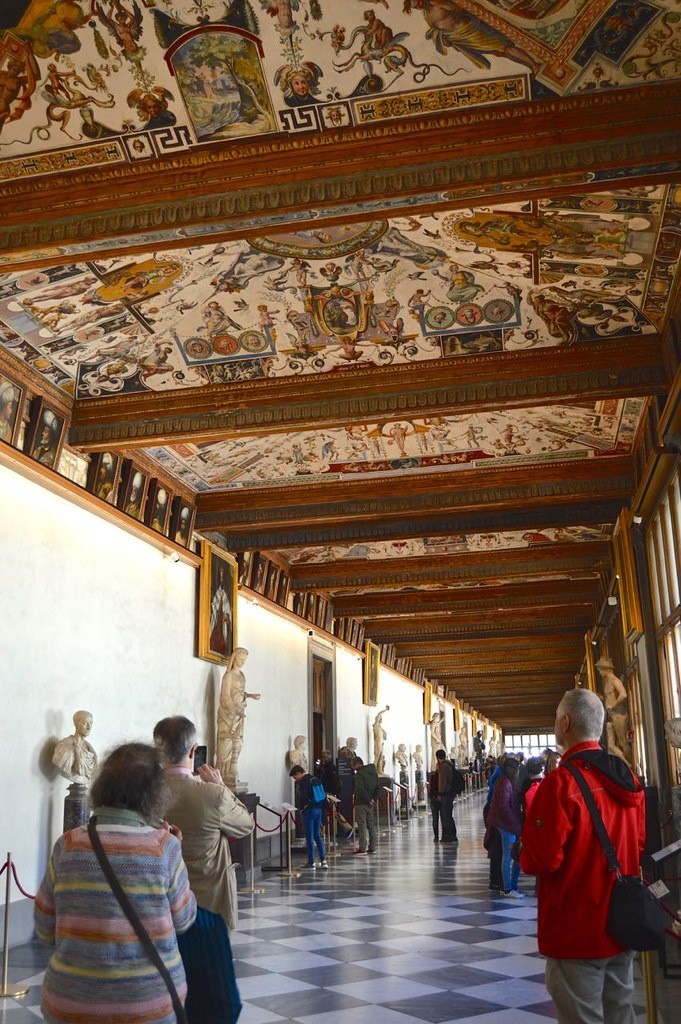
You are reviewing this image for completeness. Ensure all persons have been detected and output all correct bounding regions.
[489,737,501,757]
[342,737,358,758]
[289,764,328,870]
[34,742,196,1024]
[459,727,467,748]
[215,647,261,779]
[483,748,563,898]
[351,756,378,853]
[520,688,646,1024]
[428,749,458,843]
[288,735,307,773]
[153,715,255,1024]
[473,730,485,759]
[396,744,424,768]
[430,713,441,765]
[594,658,632,765]
[51,711,98,785]
[372,705,390,774]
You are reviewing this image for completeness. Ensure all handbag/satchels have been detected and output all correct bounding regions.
[606,875,666,952]
[177,907,242,1024]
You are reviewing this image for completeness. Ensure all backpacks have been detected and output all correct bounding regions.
[438,761,465,795]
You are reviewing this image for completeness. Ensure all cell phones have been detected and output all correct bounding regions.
[193,746,208,774]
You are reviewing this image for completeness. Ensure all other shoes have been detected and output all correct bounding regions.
[504,888,527,899]
[443,838,457,844]
[351,850,376,855]
[433,838,445,843]
[488,884,504,895]
[298,861,315,871]
[316,860,328,869]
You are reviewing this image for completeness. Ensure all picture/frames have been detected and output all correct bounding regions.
[167,496,197,548]
[88,448,124,507]
[423,680,433,725]
[198,540,239,667]
[247,551,426,684]
[0,368,28,449]
[612,506,644,644]
[26,395,71,471]
[118,458,151,523]
[453,698,461,731]
[363,641,381,707]
[145,478,174,538]
[572,627,598,696]
[473,711,502,743]
